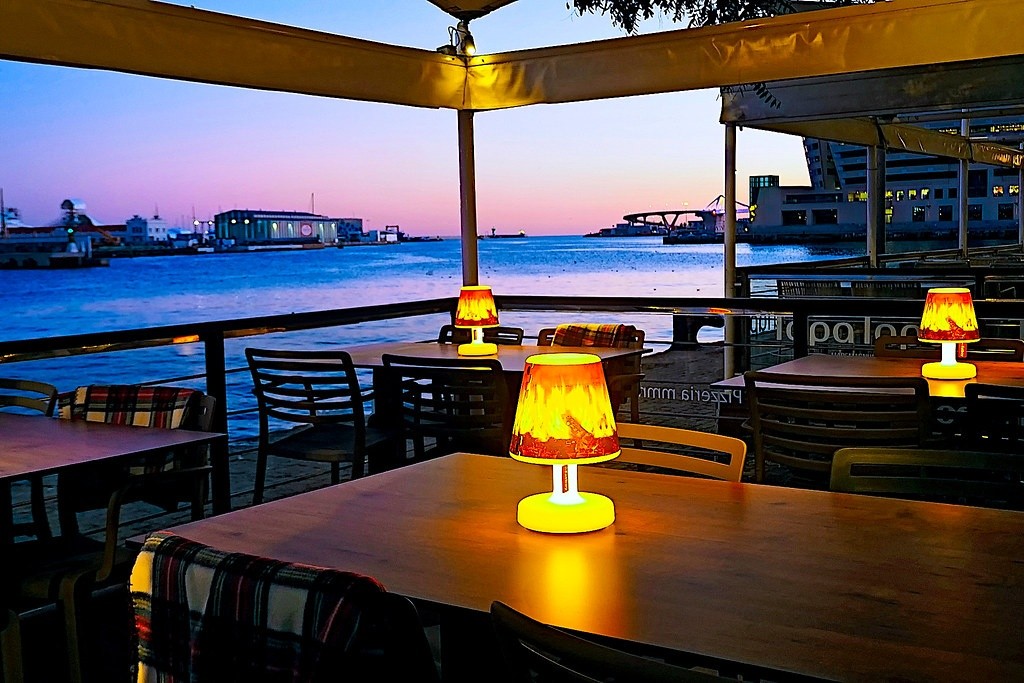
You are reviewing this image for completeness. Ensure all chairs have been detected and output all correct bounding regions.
[1,272,1024,683]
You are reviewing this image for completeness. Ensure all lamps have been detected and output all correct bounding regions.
[511,353,620,530]
[436,26,481,58]
[918,288,978,397]
[454,286,499,356]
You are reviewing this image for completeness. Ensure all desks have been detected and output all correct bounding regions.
[0,412,235,683]
[259,343,657,456]
[709,354,1024,511]
[135,454,1024,683]
